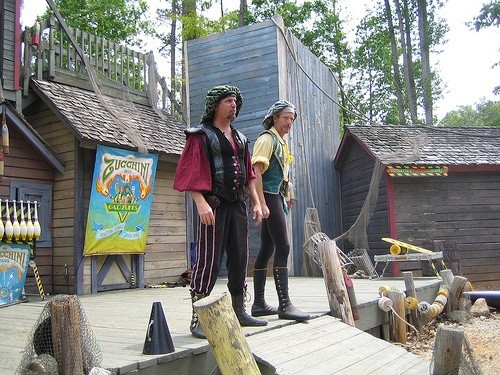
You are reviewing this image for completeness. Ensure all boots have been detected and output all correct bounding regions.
[273,266,310,320]
[251,268,278,317]
[231,293,268,326]
[190,292,207,339]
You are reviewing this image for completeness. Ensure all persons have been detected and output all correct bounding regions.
[172,85,268,339]
[250,100,310,321]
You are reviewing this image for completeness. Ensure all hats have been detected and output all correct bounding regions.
[262,100,297,130]
[204,85,242,119]
